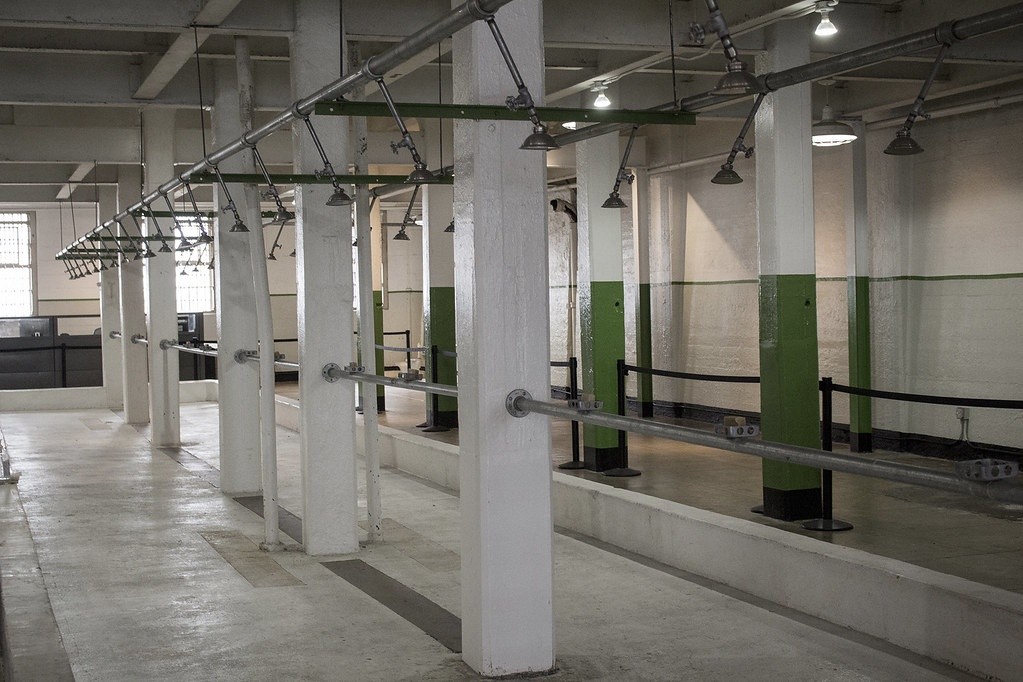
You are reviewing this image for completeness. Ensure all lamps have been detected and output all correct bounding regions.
[811,77,858,147]
[814,11,838,36]
[562,122,577,131]
[594,90,611,108]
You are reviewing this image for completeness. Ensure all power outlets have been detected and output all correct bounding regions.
[954,407,970,420]
